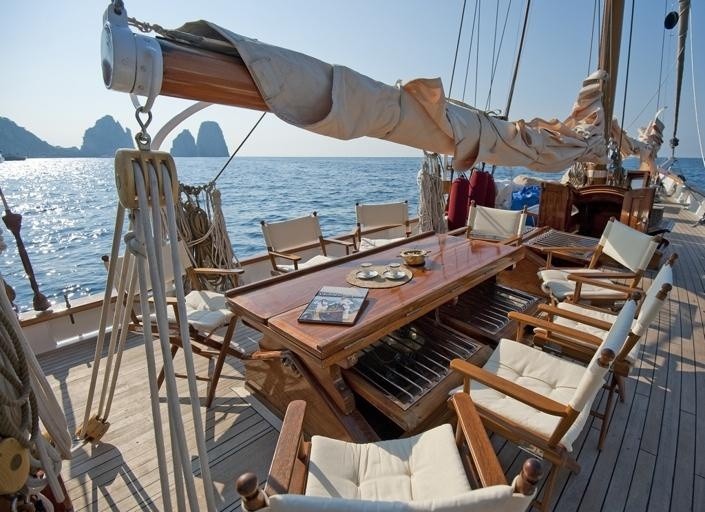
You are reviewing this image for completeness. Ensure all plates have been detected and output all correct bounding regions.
[356,270,378,279]
[383,270,406,280]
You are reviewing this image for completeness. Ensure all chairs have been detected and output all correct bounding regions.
[261,211,355,276]
[536,215,663,319]
[236,392,544,510]
[101,235,251,408]
[443,201,531,246]
[446,290,644,510]
[508,252,678,454]
[351,201,413,255]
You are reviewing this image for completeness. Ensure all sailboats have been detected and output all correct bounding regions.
[0,0,704,512]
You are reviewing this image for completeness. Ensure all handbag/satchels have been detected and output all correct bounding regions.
[510,185,540,227]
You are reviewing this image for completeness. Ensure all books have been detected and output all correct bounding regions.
[297,285,371,327]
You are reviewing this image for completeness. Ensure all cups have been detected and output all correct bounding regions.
[359,263,374,276]
[384,262,401,276]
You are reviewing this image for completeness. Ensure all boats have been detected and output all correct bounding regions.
[3,153,26,160]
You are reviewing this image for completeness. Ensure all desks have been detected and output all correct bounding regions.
[225,230,529,446]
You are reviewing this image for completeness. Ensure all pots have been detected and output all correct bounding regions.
[399,246,430,265]
